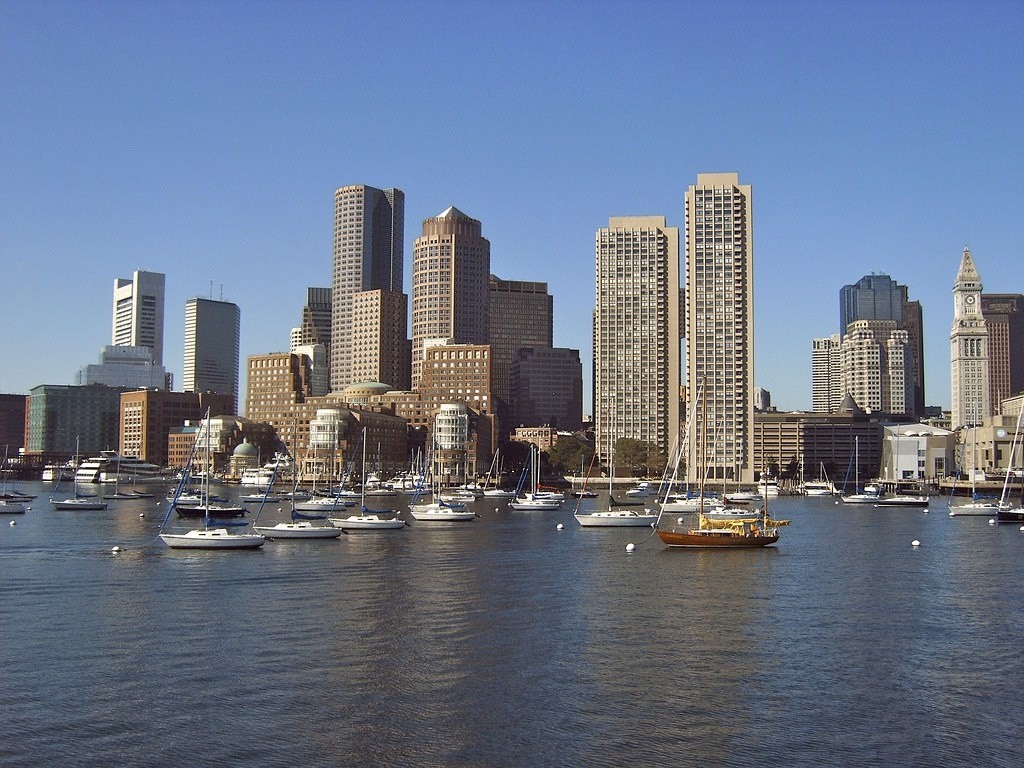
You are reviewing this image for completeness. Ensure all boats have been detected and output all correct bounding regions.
[719,490,766,500]
[625,482,657,499]
[573,454,658,527]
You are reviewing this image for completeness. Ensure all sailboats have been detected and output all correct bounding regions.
[0,444,37,514]
[801,460,836,495]
[838,436,878,504]
[92,404,517,521]
[158,406,266,549]
[997,396,1024,524]
[651,374,793,550]
[948,402,1001,515]
[48,434,156,509]
[251,417,343,539]
[510,437,564,510]
[328,426,407,531]
[874,425,930,507]
[572,454,599,497]
